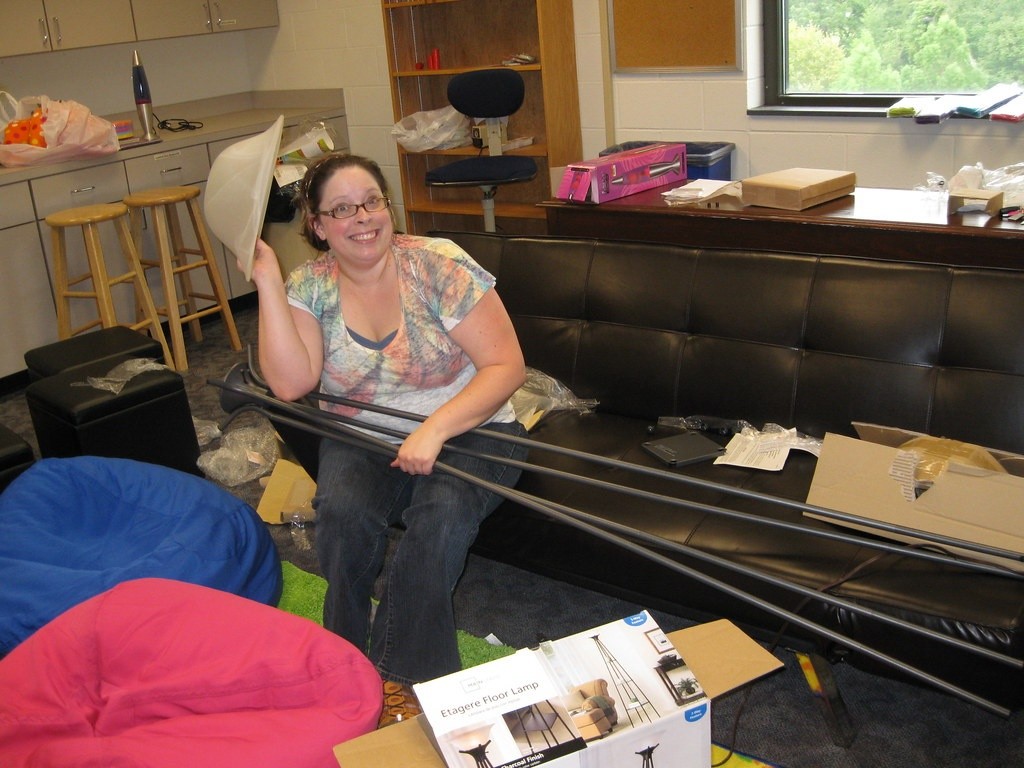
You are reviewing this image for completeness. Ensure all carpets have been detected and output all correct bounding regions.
[278,559,519,669]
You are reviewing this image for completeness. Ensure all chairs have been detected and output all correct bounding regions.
[424,68,539,233]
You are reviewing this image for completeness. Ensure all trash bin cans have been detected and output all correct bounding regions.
[598,139,737,181]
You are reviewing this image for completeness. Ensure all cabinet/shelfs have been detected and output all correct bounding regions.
[207,106,353,299]
[130,0,281,42]
[380,0,584,233]
[29,133,234,337]
[0,0,138,58]
[0,167,60,379]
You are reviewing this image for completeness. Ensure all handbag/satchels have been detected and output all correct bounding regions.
[390,106,473,154]
[0,89,120,169]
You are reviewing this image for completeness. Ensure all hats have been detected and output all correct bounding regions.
[203,115,284,283]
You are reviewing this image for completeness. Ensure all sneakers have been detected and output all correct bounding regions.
[377,678,420,730]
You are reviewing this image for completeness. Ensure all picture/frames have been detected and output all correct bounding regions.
[606,1,747,82]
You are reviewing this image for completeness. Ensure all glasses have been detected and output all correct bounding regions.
[312,196,390,219]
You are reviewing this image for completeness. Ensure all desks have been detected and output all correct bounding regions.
[535,179,1024,269]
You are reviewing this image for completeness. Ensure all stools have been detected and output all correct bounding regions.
[26,352,206,479]
[45,201,178,373]
[24,325,167,383]
[123,186,243,373]
[0,425,38,490]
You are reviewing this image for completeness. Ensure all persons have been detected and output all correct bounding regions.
[234,151,528,687]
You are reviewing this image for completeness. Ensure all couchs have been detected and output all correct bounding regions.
[219,231,1024,718]
